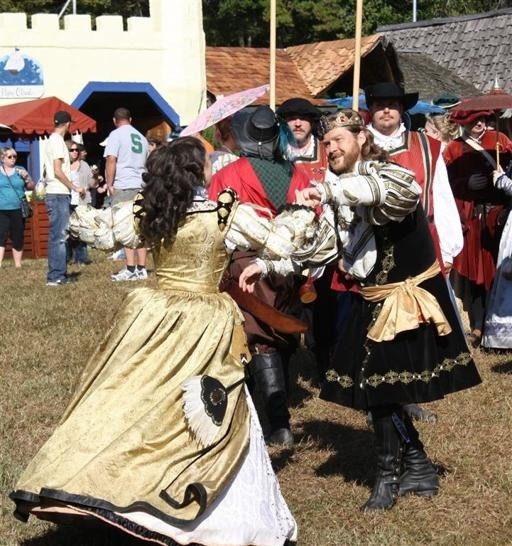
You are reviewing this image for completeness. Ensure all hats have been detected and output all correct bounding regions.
[431,93,461,108]
[54,111,77,125]
[276,97,324,119]
[360,40,420,108]
[231,104,281,159]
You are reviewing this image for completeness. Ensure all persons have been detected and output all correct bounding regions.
[45,107,150,289]
[0,146,34,269]
[10,136,322,546]
[238,109,483,511]
[203,82,512,447]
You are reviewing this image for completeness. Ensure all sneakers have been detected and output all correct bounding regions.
[134,266,149,280]
[111,265,138,281]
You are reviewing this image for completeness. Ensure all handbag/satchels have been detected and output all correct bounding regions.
[20,201,33,218]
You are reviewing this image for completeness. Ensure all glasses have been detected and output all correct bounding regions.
[7,155,17,159]
[69,148,79,153]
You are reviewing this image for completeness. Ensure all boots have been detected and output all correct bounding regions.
[252,350,295,447]
[396,423,438,498]
[361,418,402,513]
